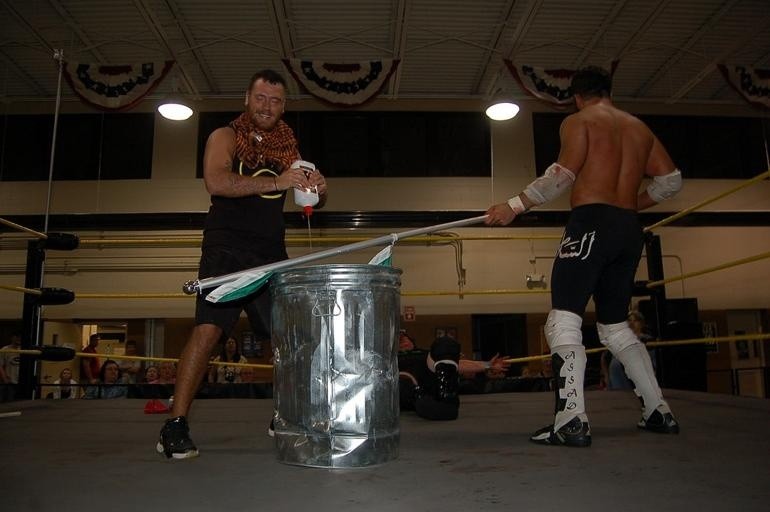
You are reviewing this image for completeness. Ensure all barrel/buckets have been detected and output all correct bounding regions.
[268,264,404,469]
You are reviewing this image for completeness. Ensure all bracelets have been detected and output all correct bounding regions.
[273,175,280,192]
[506,195,526,217]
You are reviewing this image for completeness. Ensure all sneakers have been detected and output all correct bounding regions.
[636,400,680,434]
[529,413,592,448]
[156,415,198,459]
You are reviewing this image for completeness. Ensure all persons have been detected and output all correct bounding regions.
[485,66,682,446]
[50,335,256,402]
[600,312,654,391]
[153,71,328,459]
[399,326,556,421]
[0,329,22,403]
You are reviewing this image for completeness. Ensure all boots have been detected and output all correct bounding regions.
[413,358,460,421]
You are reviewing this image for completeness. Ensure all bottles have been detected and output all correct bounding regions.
[169,396,175,411]
